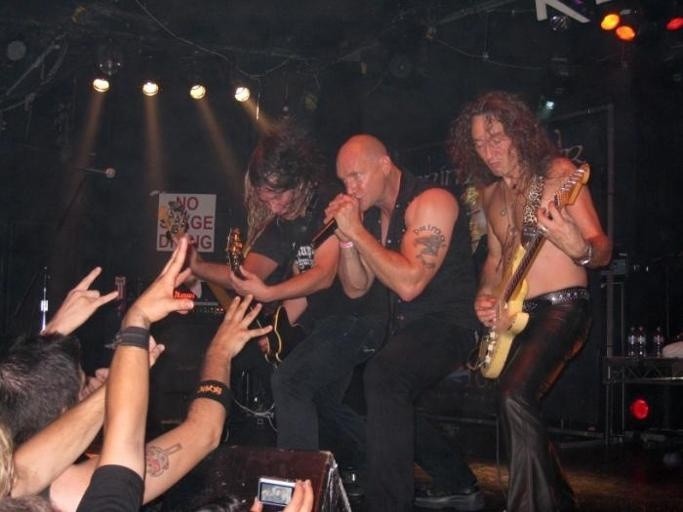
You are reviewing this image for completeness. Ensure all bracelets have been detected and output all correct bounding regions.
[573,238,594,265]
[338,240,354,249]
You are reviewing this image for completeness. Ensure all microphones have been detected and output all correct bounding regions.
[70,166,117,179]
[309,219,336,249]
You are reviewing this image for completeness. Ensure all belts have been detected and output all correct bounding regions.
[522,287,592,313]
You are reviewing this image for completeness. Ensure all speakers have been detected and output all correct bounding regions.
[226,447,352,512]
[145,309,225,431]
[502,284,631,429]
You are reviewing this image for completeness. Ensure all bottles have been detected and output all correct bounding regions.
[652,326,664,357]
[637,325,647,358]
[628,325,638,357]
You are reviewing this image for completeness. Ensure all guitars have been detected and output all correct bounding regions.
[479,164,591,380]
[224,227,306,366]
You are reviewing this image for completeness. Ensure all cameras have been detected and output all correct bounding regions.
[256,474,294,507]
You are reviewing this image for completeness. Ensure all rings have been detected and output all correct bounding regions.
[540,226,547,232]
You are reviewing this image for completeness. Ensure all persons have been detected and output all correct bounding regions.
[446,89,617,512]
[323,129,487,511]
[169,118,390,497]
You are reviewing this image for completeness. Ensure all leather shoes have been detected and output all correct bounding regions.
[414,480,487,511]
